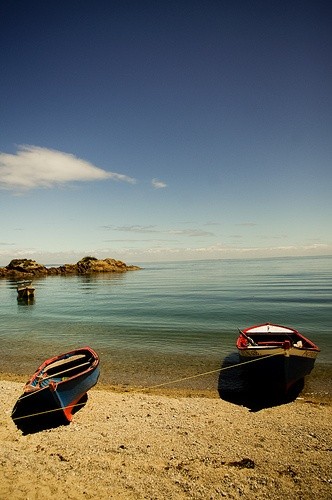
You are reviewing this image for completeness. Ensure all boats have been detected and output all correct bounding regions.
[17,278,34,299]
[11,347,100,431]
[234,322,318,390]
